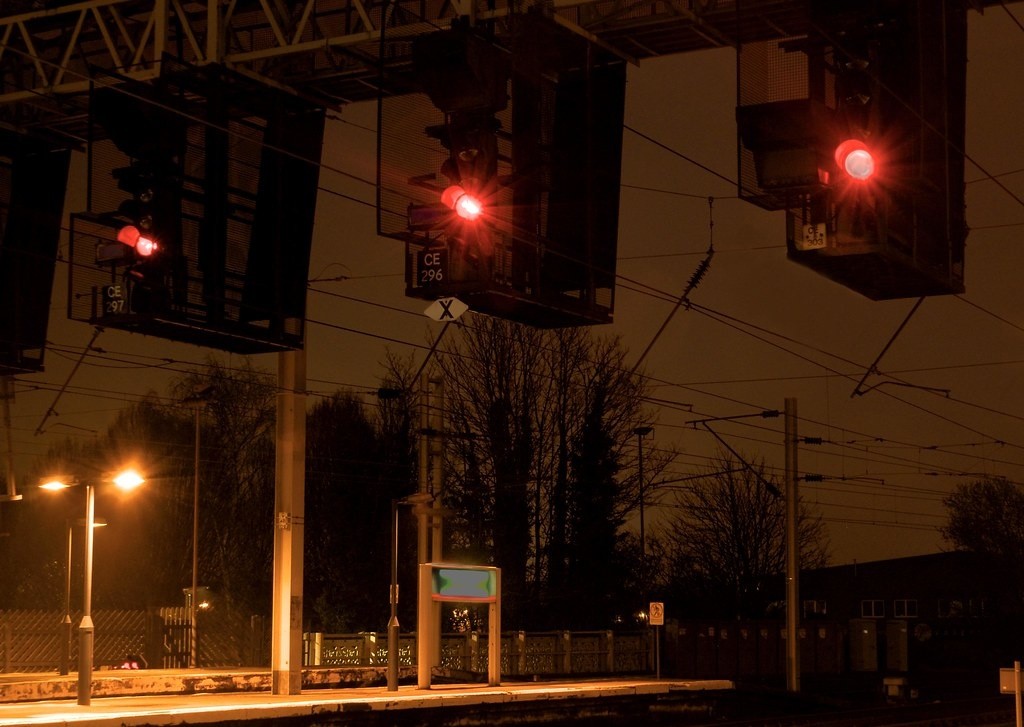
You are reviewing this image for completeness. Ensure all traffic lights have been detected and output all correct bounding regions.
[813,25,913,246]
[101,94,188,309]
[429,54,505,234]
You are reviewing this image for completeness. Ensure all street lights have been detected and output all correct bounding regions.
[178,383,216,668]
[632,426,654,579]
[58,516,107,679]
[383,492,444,695]
[38,467,145,708]
